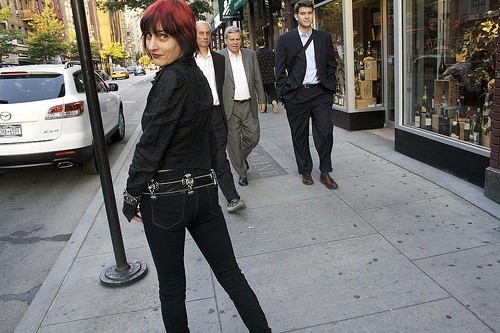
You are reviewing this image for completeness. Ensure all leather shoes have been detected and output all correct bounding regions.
[302,173,314,185]
[320,172,338,189]
[239,176,248,186]
[245,159,249,170]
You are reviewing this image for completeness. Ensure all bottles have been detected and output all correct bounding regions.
[450,92,493,145]
[366,40,372,58]
[354,63,365,98]
[439,55,446,80]
[439,90,449,136]
[334,75,345,106]
[415,86,435,131]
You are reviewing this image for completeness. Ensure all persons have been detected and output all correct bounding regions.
[217,26,266,186]
[122,0,272,333]
[193,21,246,214]
[212,44,227,52]
[334,36,343,64]
[275,0,339,190]
[255,37,279,113]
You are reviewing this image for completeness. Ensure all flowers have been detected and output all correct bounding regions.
[454,11,499,92]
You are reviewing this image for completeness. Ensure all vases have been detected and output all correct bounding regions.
[481,93,492,146]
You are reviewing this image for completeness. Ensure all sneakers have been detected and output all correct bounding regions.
[227,199,246,214]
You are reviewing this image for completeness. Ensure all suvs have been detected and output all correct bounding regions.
[0,61,125,176]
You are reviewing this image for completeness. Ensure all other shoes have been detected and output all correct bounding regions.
[272,99,279,114]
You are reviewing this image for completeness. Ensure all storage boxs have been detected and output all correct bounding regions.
[433,79,459,110]
[360,60,378,99]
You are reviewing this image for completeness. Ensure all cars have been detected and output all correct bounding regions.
[111,67,129,80]
[96,70,109,81]
[127,66,136,74]
[134,68,146,76]
